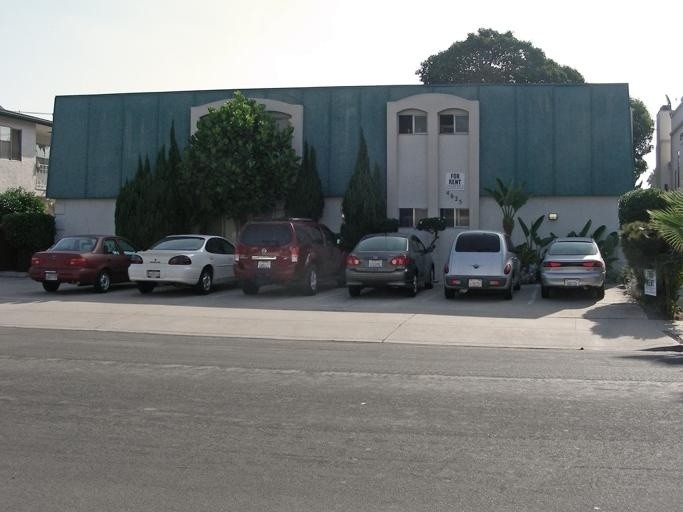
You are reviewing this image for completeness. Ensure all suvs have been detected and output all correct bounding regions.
[231,215,356,295]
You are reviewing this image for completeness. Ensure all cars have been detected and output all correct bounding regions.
[124,235,244,293]
[27,233,146,294]
[335,232,440,297]
[440,228,526,297]
[538,237,610,299]
